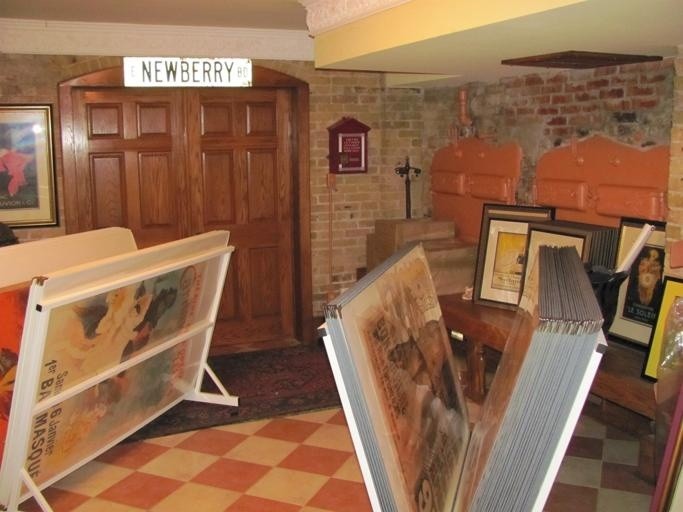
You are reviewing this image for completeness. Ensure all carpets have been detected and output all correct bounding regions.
[117,340,340,445]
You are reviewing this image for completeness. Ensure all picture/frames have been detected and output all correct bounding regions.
[469,203,683,384]
[0,103,58,231]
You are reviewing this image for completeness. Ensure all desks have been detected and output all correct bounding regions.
[437,291,656,420]
